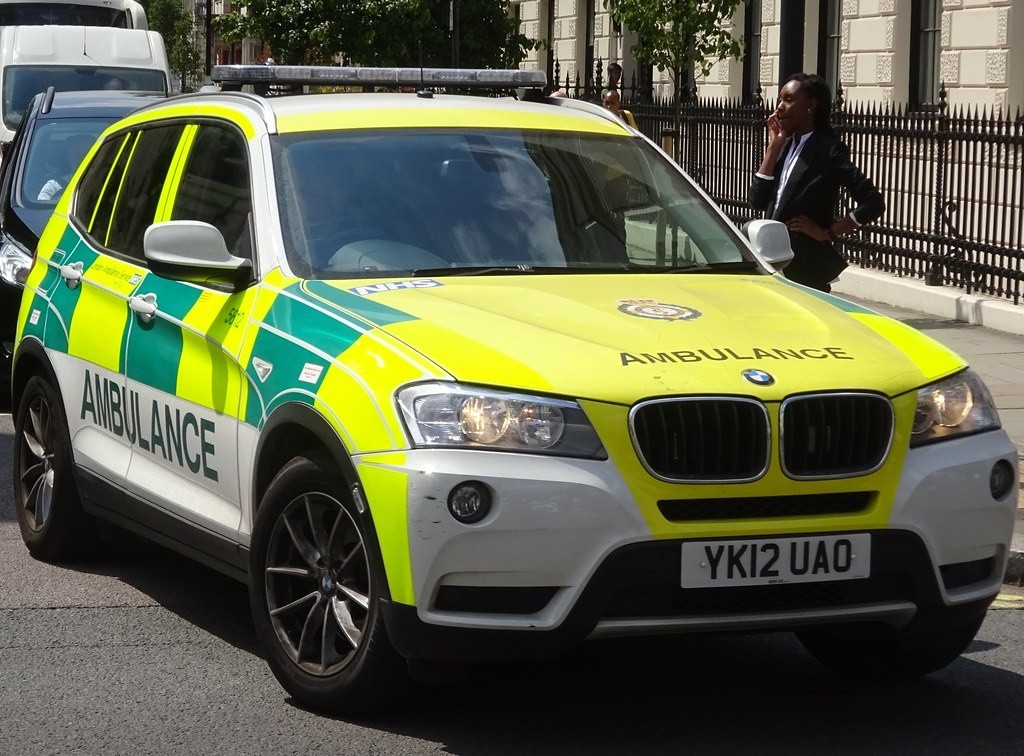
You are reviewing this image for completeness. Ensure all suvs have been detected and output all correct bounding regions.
[1,84,183,369]
[8,60,1021,711]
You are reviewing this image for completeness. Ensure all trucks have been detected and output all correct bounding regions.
[0,0,174,166]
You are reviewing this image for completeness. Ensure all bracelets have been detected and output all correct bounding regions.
[826,224,841,243]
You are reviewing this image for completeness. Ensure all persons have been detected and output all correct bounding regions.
[602,90,638,131]
[294,152,591,267]
[549,91,568,98]
[601,62,622,100]
[747,71,886,293]
[36,134,97,202]
